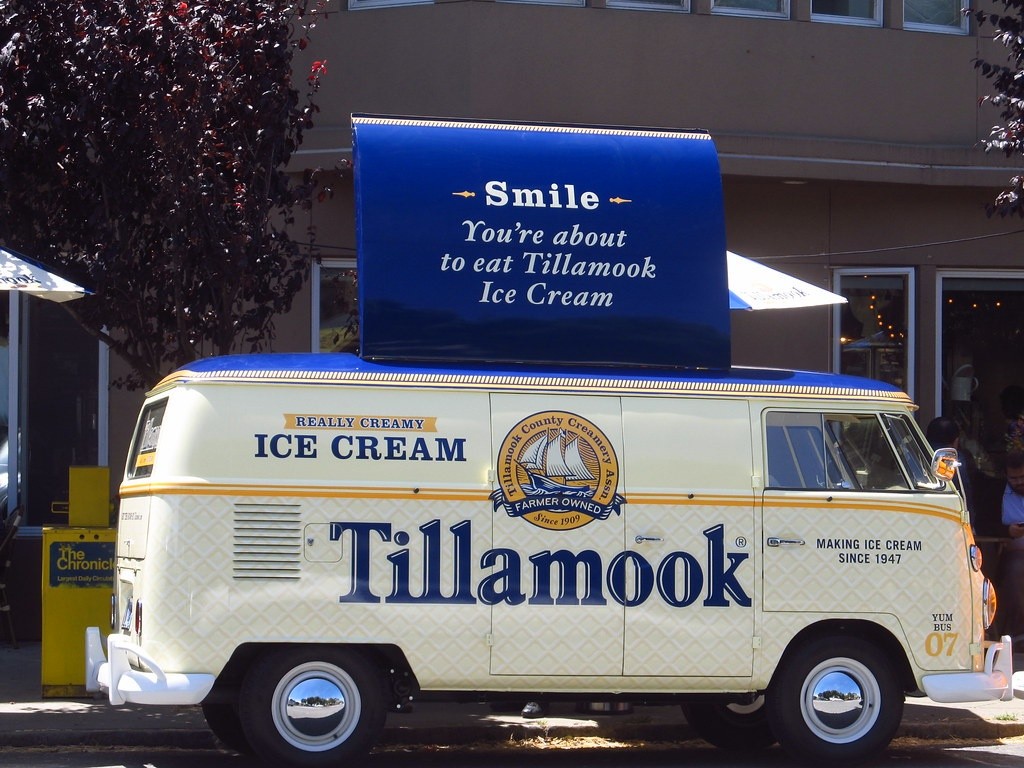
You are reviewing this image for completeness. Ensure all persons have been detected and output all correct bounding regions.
[999,384,1024,452]
[989,452,1024,653]
[925,416,979,537]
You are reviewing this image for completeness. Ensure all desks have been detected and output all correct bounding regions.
[975,535,1011,642]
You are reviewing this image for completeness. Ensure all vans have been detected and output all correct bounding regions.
[80,114,1016,768]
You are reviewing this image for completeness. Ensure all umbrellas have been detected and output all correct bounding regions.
[843,329,904,351]
[0,248,98,304]
[725,249,849,311]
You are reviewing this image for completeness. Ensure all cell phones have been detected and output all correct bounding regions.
[1017,522,1024,527]
